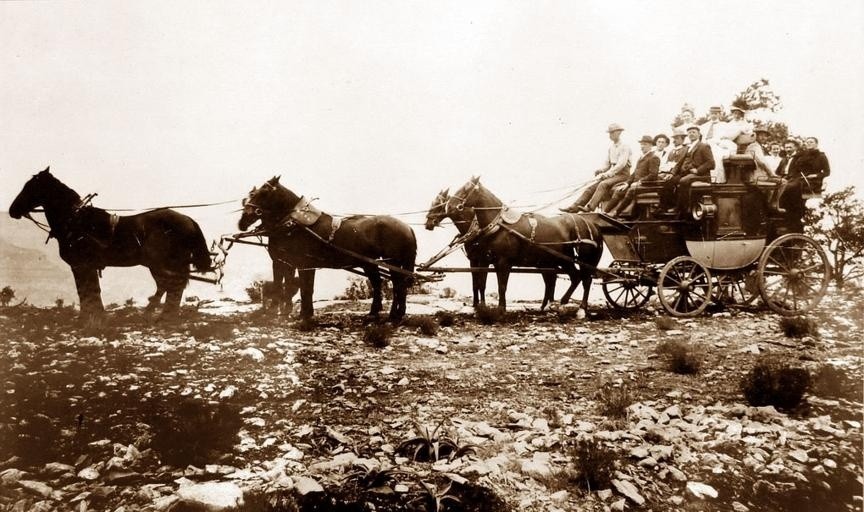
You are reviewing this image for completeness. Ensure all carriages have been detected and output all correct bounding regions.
[8,156,831,330]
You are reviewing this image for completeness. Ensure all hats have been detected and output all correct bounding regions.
[607,123,625,133]
[638,136,653,143]
[652,131,671,147]
[670,128,688,138]
[753,125,771,136]
[782,137,800,148]
[730,105,745,113]
[708,106,723,112]
[687,124,701,131]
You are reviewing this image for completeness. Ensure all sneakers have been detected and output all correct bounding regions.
[667,207,680,213]
[606,210,618,220]
[558,207,577,213]
[577,205,591,213]
[772,206,786,214]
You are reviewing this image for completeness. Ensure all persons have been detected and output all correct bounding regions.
[559,101,831,219]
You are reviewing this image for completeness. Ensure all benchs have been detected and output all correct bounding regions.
[599,154,826,220]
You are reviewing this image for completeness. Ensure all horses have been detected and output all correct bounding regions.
[447,173,603,316]
[425,187,557,311]
[237,185,383,319]
[243,173,418,328]
[8,165,212,323]
